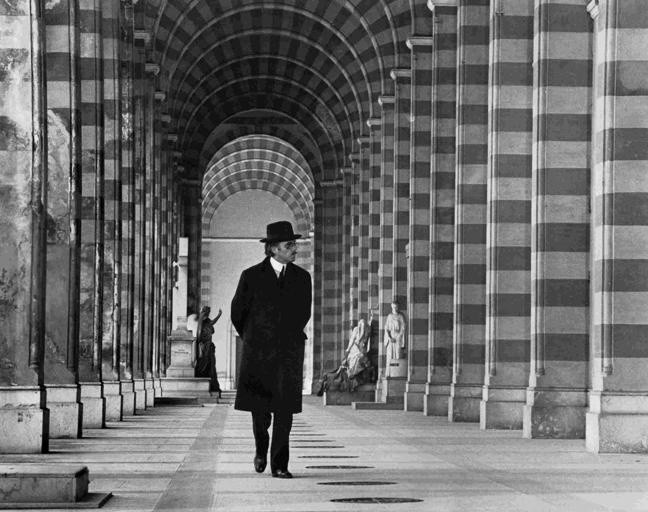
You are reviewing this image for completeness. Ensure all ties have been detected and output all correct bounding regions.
[279,265,284,282]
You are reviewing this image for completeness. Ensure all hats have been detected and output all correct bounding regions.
[259,221,302,242]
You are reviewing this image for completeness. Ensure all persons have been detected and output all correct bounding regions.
[346,319,372,379]
[383,301,407,360]
[197,305,224,341]
[231,221,312,479]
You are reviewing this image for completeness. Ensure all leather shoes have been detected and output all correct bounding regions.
[272,469,292,478]
[254,455,267,472]
